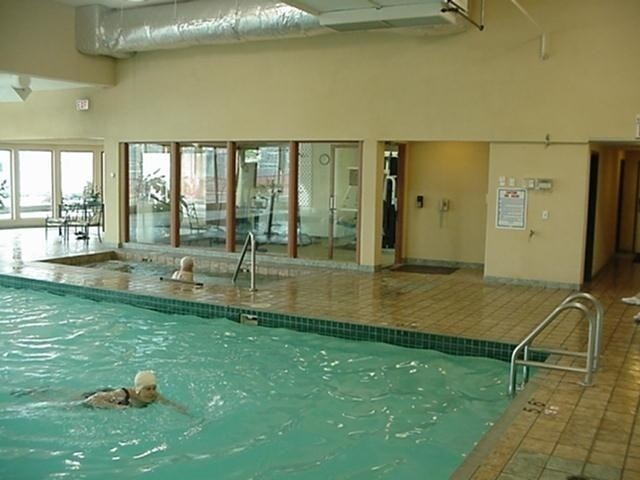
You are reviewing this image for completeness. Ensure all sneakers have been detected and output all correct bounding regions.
[621,297,640,305]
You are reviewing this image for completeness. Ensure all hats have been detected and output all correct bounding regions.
[134,372,156,395]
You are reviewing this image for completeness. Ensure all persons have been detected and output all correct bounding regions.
[9,370,210,422]
[619,287,640,325]
[170,255,195,283]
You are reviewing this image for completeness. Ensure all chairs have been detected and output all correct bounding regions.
[188,199,217,247]
[43,203,105,246]
